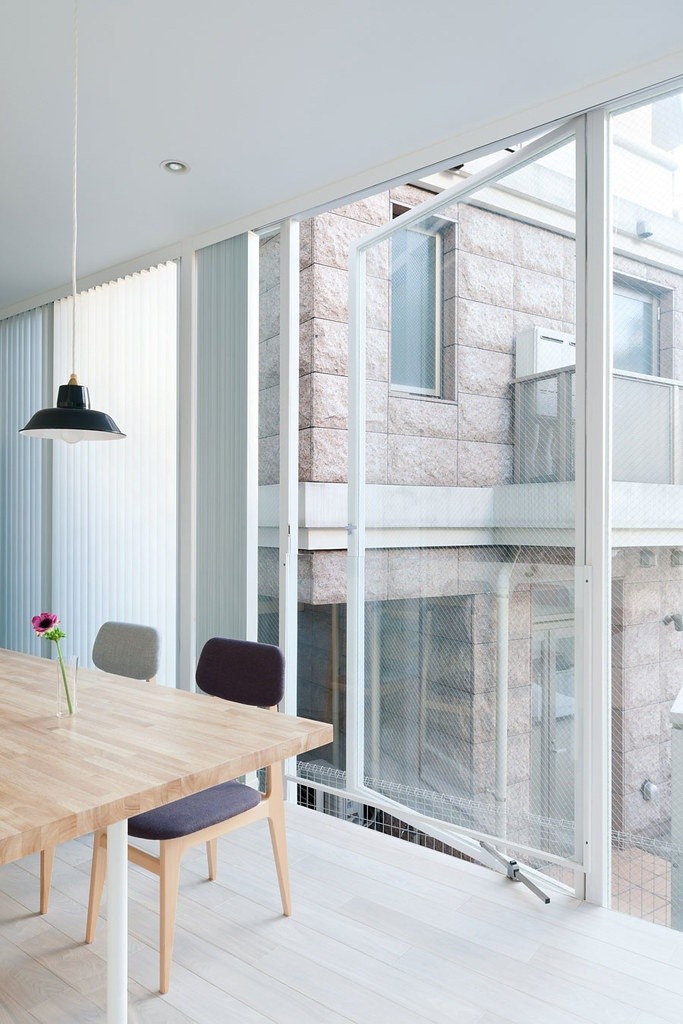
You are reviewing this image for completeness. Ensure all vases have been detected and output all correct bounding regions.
[56,655,80,717]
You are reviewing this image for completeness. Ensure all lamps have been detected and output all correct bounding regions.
[19,18,128,445]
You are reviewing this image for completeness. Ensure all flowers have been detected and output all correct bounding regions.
[31,612,73,715]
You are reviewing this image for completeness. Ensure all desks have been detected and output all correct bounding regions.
[0,647,335,1024]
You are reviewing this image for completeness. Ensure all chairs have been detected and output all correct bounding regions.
[38,621,162,915]
[83,637,293,994]
